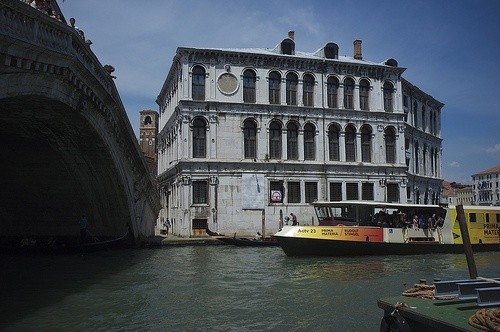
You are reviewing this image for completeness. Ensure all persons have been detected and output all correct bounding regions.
[369,210,444,230]
[78,29,93,49]
[163,218,171,236]
[104,63,117,79]
[23,0,62,22]
[290,212,297,226]
[69,18,79,33]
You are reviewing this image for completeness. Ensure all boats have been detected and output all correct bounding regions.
[268,199,500,257]
